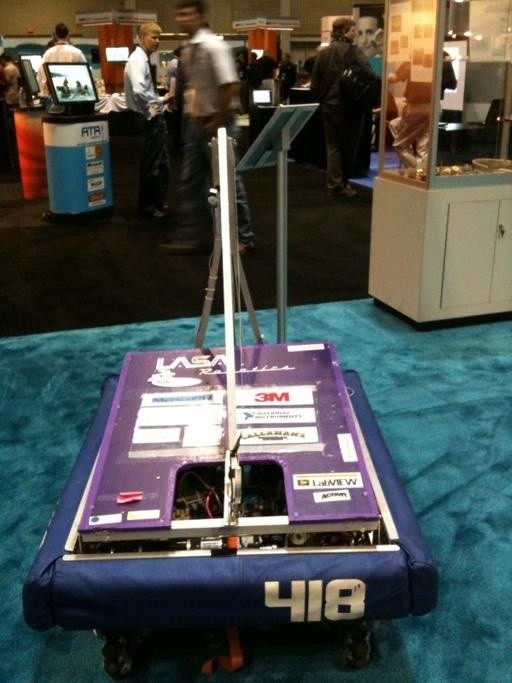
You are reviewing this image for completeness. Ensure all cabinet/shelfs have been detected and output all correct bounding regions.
[368,0,512,332]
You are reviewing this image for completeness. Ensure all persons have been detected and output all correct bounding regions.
[167,46,184,150]
[309,17,381,201]
[387,48,458,176]
[125,22,174,217]
[160,1,253,253]
[353,16,387,60]
[241,50,303,106]
[0,22,88,108]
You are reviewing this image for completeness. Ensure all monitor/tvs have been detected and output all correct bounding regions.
[251,49,265,60]
[44,61,100,104]
[18,58,41,96]
[252,89,271,105]
[105,47,130,63]
[20,52,44,69]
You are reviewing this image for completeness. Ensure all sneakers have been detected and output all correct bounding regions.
[138,203,179,218]
[327,184,357,197]
[238,239,256,255]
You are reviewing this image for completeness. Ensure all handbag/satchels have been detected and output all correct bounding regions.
[338,45,379,113]
